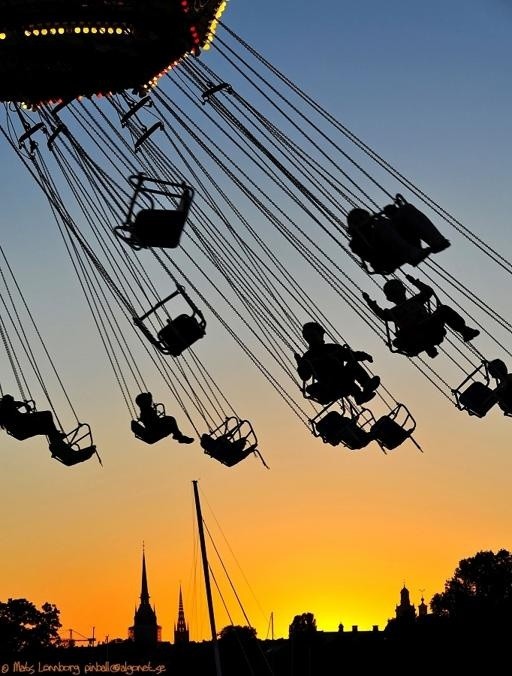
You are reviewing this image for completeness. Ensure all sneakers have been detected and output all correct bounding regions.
[355,376,380,405]
[423,327,481,358]
[173,433,195,443]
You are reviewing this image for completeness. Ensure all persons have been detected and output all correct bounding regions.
[485,358,511,419]
[133,391,195,445]
[361,272,482,359]
[344,202,453,275]
[0,394,67,446]
[292,320,381,406]
